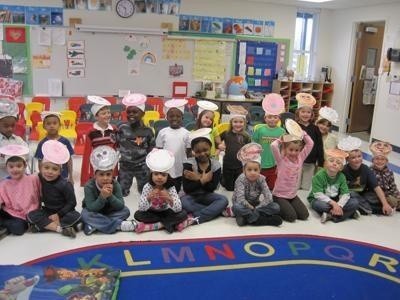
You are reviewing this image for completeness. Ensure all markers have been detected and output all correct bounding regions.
[158,96,165,97]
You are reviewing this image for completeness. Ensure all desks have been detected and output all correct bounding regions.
[192,95,263,119]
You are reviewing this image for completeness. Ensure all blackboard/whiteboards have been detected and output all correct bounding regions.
[30,24,237,100]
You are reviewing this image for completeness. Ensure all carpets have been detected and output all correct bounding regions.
[0,233,400,299]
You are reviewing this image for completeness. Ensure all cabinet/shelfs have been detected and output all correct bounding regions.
[271,79,334,125]
[32,95,197,119]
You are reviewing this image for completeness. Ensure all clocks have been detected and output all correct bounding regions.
[116,0,135,18]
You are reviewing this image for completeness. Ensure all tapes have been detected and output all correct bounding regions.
[392,73,399,81]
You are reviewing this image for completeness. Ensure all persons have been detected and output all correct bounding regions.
[77,106,119,209]
[231,160,284,227]
[25,160,81,239]
[80,168,136,236]
[34,114,74,191]
[215,116,253,192]
[269,131,315,223]
[155,107,193,194]
[306,156,362,224]
[134,169,188,234]
[316,118,341,162]
[0,156,42,239]
[251,115,287,191]
[189,110,221,161]
[365,155,400,212]
[340,149,393,215]
[116,105,154,199]
[0,113,31,178]
[285,105,325,191]
[177,137,233,234]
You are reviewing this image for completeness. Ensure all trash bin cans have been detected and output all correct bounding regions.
[282,112,294,126]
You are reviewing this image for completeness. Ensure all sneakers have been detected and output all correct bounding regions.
[266,214,283,227]
[27,222,40,233]
[62,227,76,239]
[173,212,195,232]
[120,219,137,232]
[349,209,361,219]
[320,211,333,223]
[372,206,384,216]
[221,205,236,218]
[134,222,145,234]
[83,222,97,236]
[235,214,248,227]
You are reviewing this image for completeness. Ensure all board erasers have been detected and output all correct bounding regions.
[147,95,154,97]
[112,95,119,97]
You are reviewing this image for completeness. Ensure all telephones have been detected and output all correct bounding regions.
[387,48,400,62]
